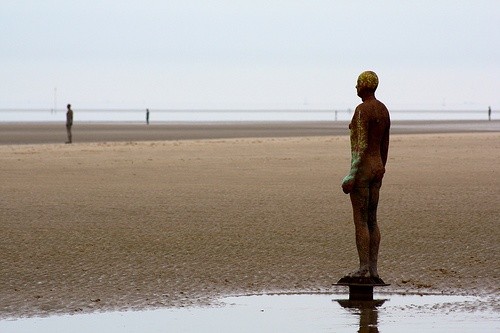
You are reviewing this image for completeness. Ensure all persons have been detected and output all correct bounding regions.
[66,103,73,144]
[338,69,391,284]
[146,108,149,124]
[489,105,492,120]
